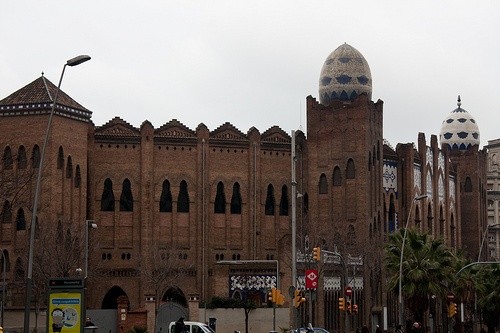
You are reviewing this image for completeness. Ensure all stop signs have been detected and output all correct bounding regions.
[344,285,353,296]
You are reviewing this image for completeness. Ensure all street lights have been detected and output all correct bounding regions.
[22,52,91,332]
[399,194,428,333]
[475,222,500,333]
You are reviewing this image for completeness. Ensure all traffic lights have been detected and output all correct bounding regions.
[338,298,346,310]
[346,301,352,315]
[268,288,276,303]
[275,289,284,305]
[294,290,302,309]
[448,303,459,318]
[312,247,321,262]
[353,304,358,314]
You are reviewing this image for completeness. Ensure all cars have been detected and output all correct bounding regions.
[168,322,216,333]
[293,326,329,333]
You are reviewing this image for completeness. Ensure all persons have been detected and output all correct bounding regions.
[356,322,363,333]
[173,321,182,333]
[413,321,423,333]
[307,322,316,333]
[86,317,96,328]
[376,325,384,333]
[362,322,371,333]
[397,324,405,333]
